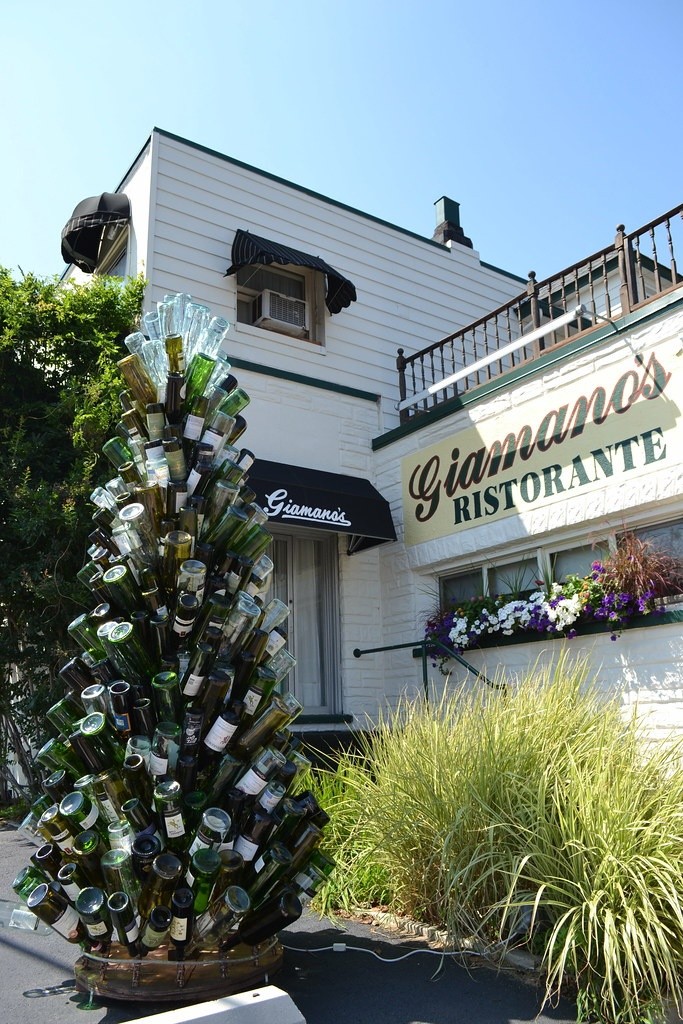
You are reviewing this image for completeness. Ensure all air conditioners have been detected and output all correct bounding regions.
[247,291,311,338]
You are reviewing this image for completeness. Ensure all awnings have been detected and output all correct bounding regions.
[223,225,359,317]
[60,192,132,274]
[245,457,398,556]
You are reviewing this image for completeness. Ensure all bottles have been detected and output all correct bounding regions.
[12,292,336,960]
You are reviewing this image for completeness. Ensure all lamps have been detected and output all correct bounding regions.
[392,304,587,412]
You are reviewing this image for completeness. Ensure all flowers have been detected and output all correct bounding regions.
[413,547,663,677]
